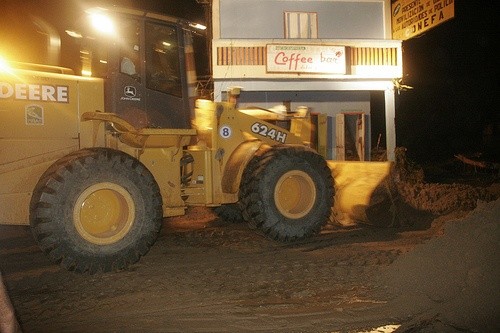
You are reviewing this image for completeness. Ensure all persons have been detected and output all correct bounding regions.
[120,47,140,78]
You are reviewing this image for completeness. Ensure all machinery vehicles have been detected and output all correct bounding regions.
[0,2,402,274]
